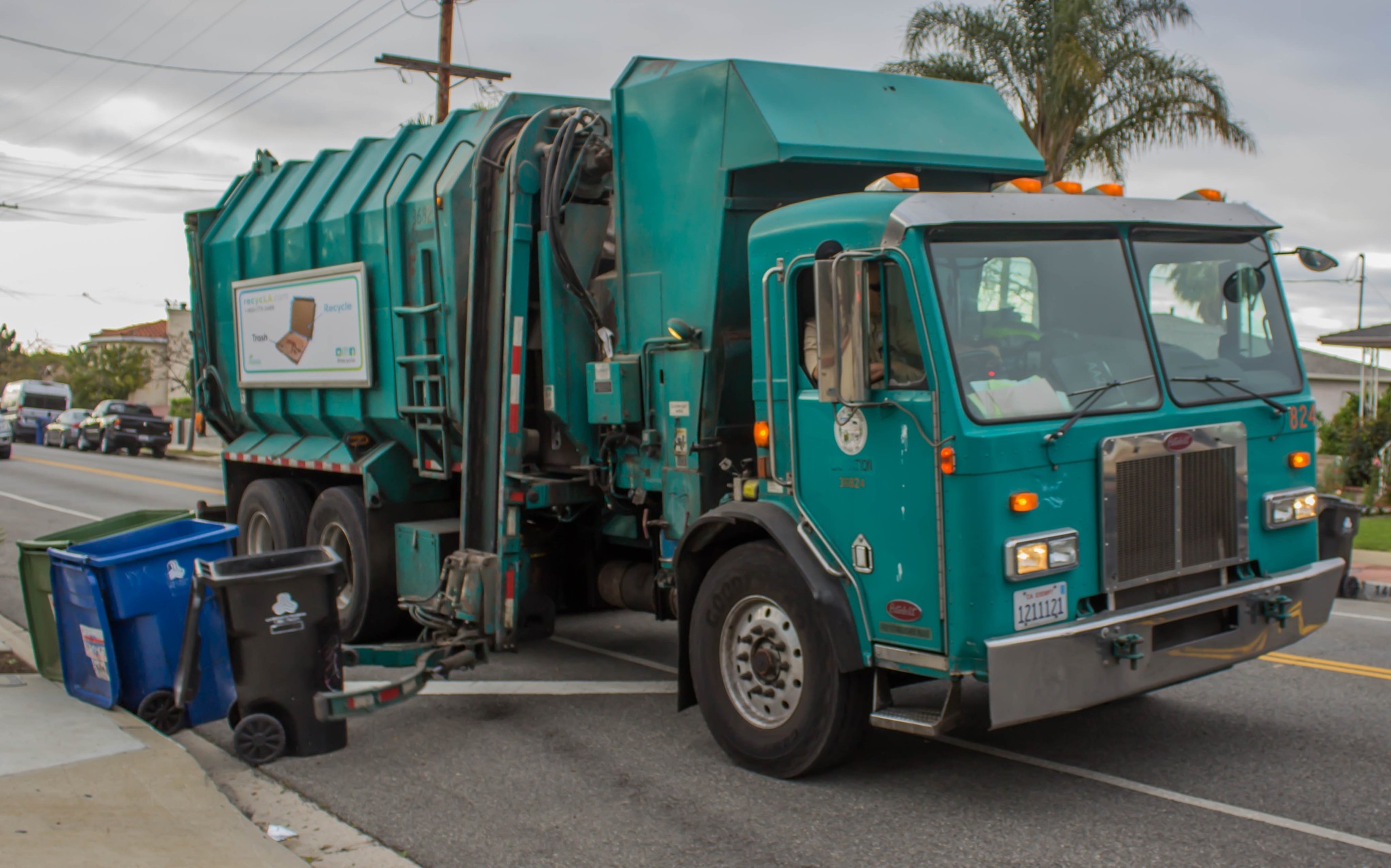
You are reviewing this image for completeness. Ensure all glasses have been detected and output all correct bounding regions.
[869,282,887,291]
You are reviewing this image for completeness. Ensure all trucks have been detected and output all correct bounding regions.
[0,379,72,459]
[184,53,1347,780]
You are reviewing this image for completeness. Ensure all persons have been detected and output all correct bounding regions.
[802,266,925,383]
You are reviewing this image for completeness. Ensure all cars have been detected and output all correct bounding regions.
[43,399,173,459]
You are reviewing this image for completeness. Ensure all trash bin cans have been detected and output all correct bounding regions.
[46,518,242,737]
[1315,494,1362,598]
[196,542,348,764]
[15,507,196,684]
